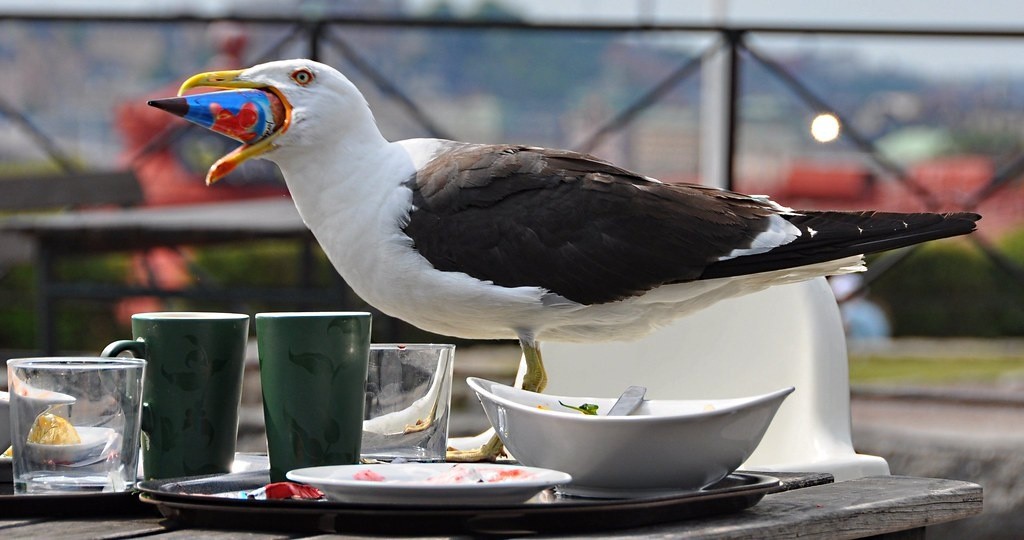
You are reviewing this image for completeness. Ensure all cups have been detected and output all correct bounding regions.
[358,344,455,464]
[99,313,249,480]
[255,313,371,484]
[7,356,146,496]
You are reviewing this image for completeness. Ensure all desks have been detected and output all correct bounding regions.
[0,196,395,348]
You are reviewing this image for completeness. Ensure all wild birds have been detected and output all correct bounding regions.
[175,56,984,461]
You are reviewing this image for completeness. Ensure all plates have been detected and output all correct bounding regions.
[286,463,573,503]
[0,457,13,482]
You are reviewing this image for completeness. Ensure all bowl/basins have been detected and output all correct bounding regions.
[466,377,796,499]
[0,390,10,455]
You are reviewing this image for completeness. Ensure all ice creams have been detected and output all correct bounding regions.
[149,90,284,145]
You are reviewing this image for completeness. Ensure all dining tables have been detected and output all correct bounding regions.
[0,477,985,540]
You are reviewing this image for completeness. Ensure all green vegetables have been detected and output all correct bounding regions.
[559,400,598,416]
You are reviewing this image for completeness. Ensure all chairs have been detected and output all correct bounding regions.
[507,271,889,484]
